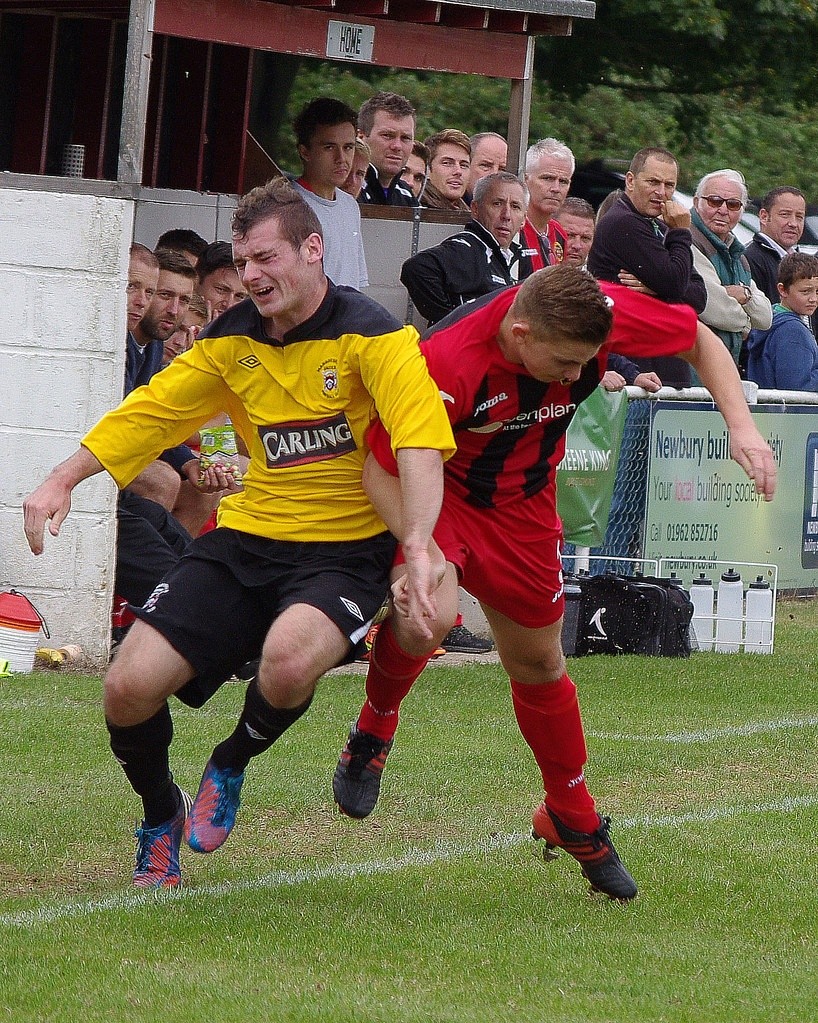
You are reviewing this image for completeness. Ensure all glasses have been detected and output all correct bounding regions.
[701,195,744,212]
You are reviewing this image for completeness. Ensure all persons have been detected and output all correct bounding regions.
[340,138,371,200]
[332,265,776,903]
[399,171,534,653]
[400,140,433,202]
[462,131,508,207]
[22,175,459,886]
[520,137,818,576]
[356,92,419,206]
[292,98,369,292]
[115,230,249,608]
[420,128,470,210]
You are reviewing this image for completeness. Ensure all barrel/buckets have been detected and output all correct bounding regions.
[0,589,51,675]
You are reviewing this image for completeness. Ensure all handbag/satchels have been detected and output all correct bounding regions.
[576,573,694,658]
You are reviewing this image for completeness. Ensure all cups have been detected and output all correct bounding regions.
[62,143,85,176]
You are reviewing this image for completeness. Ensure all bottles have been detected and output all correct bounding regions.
[743,574,773,654]
[664,571,684,592]
[714,568,743,654]
[689,572,715,653]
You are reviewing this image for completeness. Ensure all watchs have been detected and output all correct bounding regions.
[744,288,750,303]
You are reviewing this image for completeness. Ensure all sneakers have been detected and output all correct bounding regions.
[181,749,245,854]
[531,799,637,903]
[365,603,446,659]
[440,625,492,653]
[133,783,195,888]
[332,719,395,819]
[110,636,121,662]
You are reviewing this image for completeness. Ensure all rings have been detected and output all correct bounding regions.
[639,282,641,286]
[621,380,625,382]
